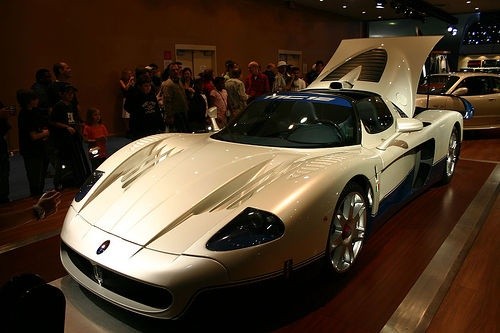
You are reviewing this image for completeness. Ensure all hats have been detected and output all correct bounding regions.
[59,83,75,91]
[277,61,289,67]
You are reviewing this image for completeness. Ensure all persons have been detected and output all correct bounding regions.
[337,108,371,144]
[83,106,109,168]
[51,83,94,193]
[0,104,12,205]
[48,61,82,128]
[116,55,323,140]
[14,69,58,200]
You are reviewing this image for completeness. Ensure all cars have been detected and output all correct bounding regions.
[414,72,500,130]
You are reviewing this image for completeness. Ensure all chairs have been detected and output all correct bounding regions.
[281,100,321,126]
[338,101,379,137]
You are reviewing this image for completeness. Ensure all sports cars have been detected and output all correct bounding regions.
[60,35,465,322]
